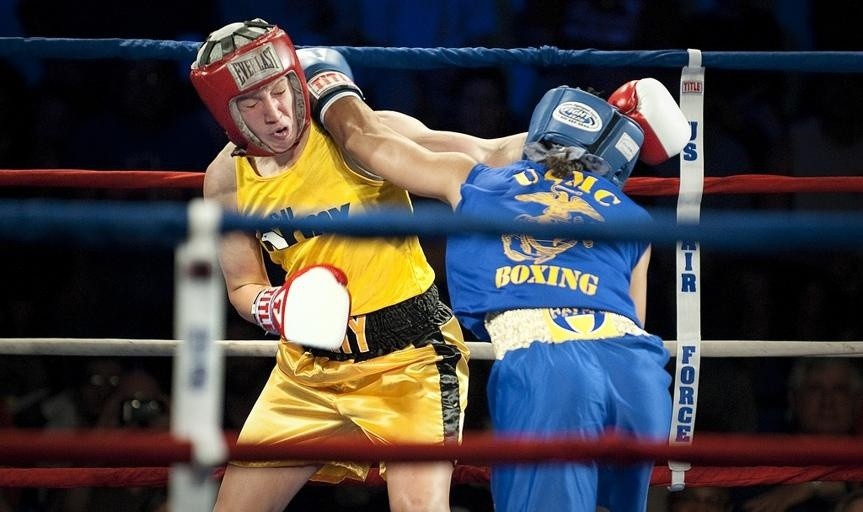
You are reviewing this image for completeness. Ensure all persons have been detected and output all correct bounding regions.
[189,18,693,512]
[295,46,673,512]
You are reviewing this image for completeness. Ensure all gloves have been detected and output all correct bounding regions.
[292,44,365,126]
[252,265,353,351]
[607,77,692,164]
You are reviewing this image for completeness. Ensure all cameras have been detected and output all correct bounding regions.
[121,393,166,427]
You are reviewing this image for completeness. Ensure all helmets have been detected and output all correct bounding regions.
[521,85,645,187]
[189,17,309,157]
